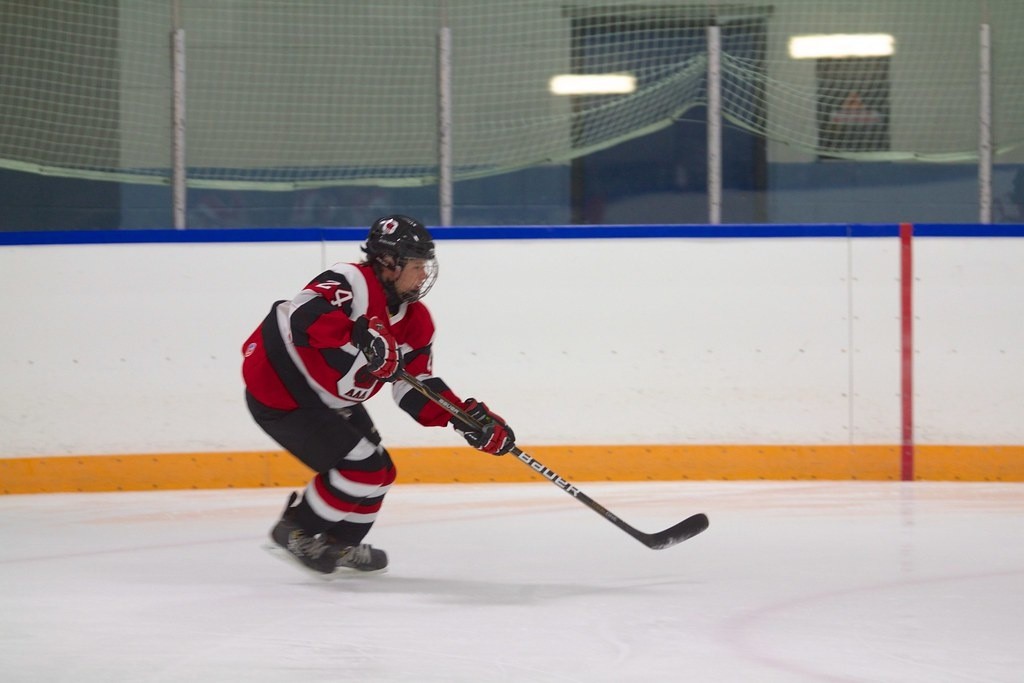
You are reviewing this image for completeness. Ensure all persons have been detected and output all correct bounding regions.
[241,214,515,573]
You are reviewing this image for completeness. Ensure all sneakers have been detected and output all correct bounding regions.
[315,532,389,575]
[263,492,336,581]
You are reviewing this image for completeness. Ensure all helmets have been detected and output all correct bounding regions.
[366,214,438,304]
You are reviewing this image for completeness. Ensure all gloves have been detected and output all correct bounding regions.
[350,314,404,383]
[453,398,515,456]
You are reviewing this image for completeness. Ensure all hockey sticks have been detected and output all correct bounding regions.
[364,346,709,550]
